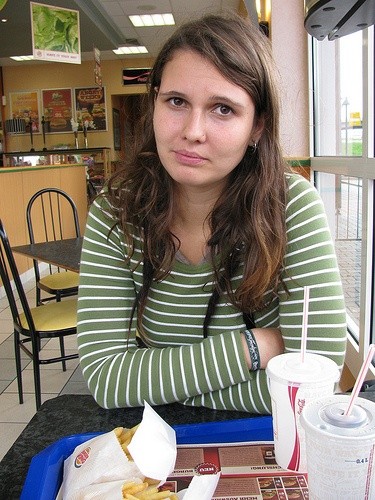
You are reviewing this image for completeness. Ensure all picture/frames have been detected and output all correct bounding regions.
[112,107,121,151]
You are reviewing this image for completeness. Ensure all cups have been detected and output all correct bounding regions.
[265,352,341,474]
[300,394,375,500]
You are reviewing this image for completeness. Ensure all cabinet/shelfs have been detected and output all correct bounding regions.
[0,147,112,198]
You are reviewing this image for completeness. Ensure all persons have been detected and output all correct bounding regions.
[77,14,347,415]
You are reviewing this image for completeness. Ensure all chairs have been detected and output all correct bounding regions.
[0,219,78,411]
[27,188,81,307]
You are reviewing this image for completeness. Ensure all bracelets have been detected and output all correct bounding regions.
[241,329,260,370]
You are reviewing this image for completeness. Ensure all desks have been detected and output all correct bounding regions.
[0,394,272,499]
[10,237,84,273]
